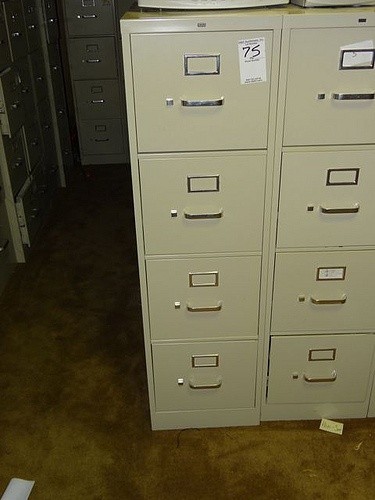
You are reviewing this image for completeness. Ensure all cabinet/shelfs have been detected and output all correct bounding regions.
[1,2,375,432]
